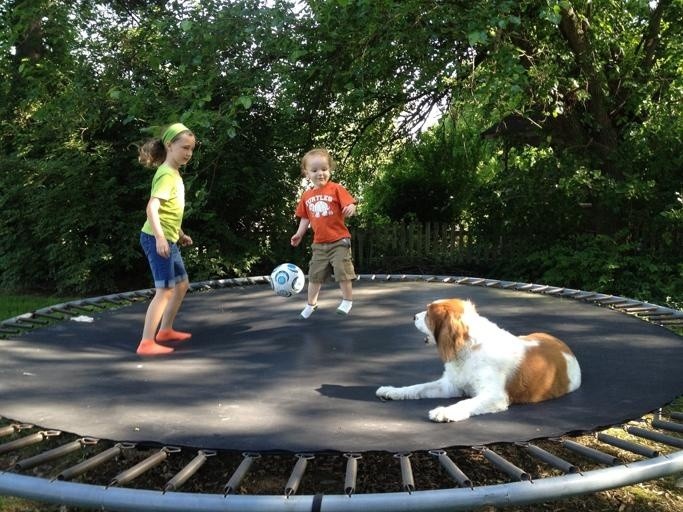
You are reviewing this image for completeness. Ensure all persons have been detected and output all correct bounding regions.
[289,148,357,321]
[136,122,196,357]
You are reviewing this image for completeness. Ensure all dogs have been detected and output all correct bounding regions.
[375,297,582,423]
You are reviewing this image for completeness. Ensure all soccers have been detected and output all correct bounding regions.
[269,262,305,297]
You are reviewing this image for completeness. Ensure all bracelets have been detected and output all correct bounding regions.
[179,233,185,240]
